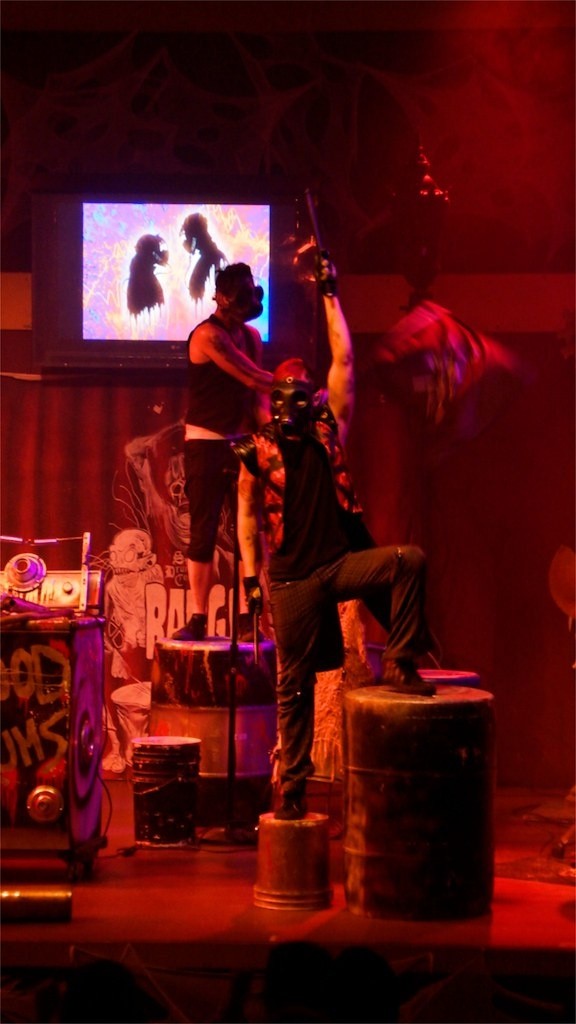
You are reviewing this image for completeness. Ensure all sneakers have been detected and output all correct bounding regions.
[380,653,436,695]
[274,798,307,820]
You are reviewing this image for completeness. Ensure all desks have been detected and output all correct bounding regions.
[157,637,273,845]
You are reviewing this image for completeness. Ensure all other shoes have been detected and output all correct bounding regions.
[171,613,206,641]
[237,615,263,642]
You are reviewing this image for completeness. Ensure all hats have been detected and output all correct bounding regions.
[271,357,316,390]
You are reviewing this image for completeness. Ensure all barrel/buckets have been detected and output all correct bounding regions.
[345,685,495,924]
[130,735,201,849]
[151,636,275,844]
[253,812,331,910]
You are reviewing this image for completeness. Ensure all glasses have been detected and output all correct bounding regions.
[270,388,313,410]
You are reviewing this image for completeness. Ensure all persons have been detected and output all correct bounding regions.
[173,264,274,641]
[236,245,436,819]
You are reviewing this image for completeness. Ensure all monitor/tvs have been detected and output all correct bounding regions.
[29,191,319,375]
